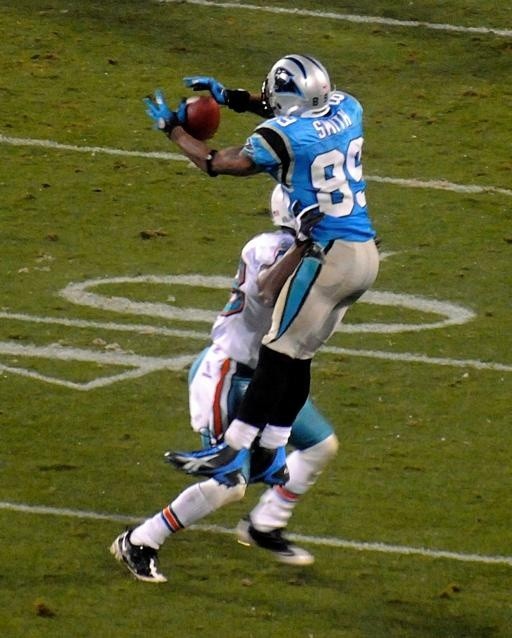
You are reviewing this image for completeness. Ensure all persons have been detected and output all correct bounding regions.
[106,181,383,584]
[143,51,381,488]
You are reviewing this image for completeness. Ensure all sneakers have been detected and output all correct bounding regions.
[250,438,289,488]
[163,427,250,489]
[234,514,315,565]
[109,526,167,583]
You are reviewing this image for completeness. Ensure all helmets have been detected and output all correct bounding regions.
[271,183,299,231]
[261,52,331,116]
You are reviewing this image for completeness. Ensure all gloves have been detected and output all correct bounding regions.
[183,77,251,112]
[142,88,187,137]
[293,202,324,245]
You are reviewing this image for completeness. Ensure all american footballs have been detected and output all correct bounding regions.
[182,96,220,141]
